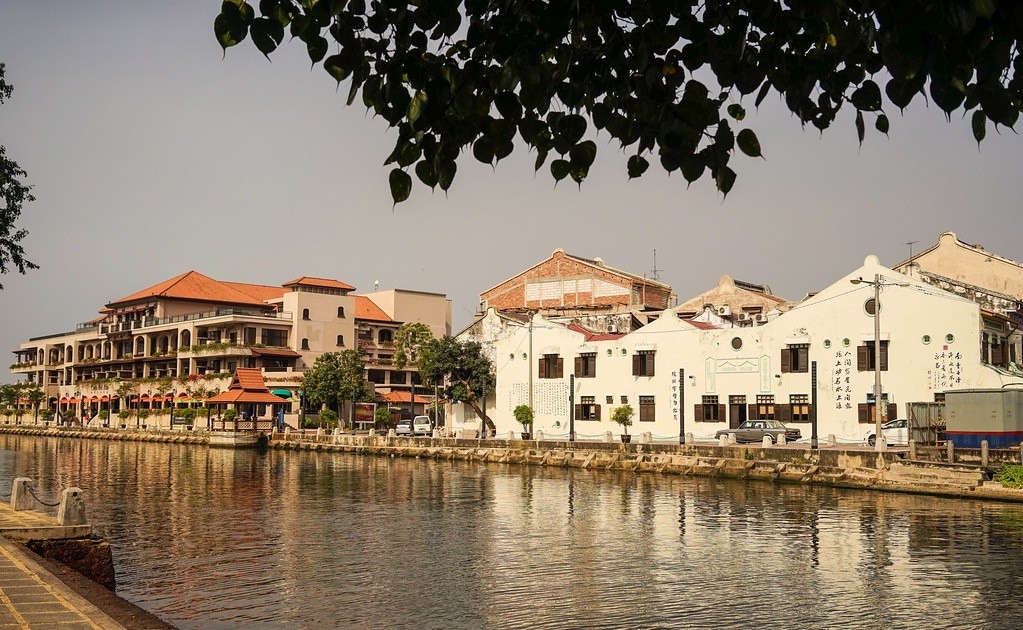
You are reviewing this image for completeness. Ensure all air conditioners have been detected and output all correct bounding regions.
[736,312,750,322]
[605,324,618,334]
[754,313,768,323]
[716,304,732,317]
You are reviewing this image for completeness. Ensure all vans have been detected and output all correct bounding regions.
[413,416,435,435]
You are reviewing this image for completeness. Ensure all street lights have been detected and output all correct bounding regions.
[849,273,910,454]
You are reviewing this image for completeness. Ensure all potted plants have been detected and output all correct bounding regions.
[512,404,535,441]
[609,404,636,444]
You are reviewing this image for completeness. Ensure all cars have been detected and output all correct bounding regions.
[865,418,909,447]
[714,420,803,444]
[396,419,411,436]
